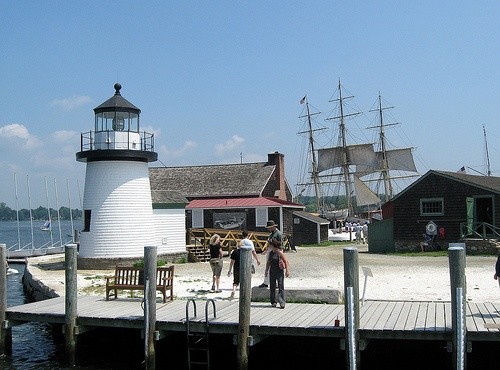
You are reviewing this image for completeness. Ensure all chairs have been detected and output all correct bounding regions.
[423,235,434,252]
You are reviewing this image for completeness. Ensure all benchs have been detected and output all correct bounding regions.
[105,264,174,303]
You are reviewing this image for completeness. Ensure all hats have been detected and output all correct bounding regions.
[265,220,278,228]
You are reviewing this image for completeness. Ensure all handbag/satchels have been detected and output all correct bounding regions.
[278,250,289,269]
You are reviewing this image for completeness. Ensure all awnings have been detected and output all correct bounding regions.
[185,196,305,210]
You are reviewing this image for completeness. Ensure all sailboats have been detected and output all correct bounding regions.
[288,80,433,229]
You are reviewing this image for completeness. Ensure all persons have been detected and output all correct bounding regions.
[236,229,260,268]
[332,218,371,244]
[419,233,432,252]
[264,238,290,309]
[208,234,224,294]
[494,253,500,287]
[258,219,285,289]
[227,243,242,299]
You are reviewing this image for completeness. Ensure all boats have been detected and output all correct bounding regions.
[219,216,246,229]
[40,218,52,231]
[66,230,82,237]
[6,259,20,275]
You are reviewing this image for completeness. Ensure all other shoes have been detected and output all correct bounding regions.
[259,283,268,288]
[231,291,235,299]
[216,289,222,293]
[211,286,215,290]
[280,303,285,308]
[276,287,279,288]
[272,303,276,307]
